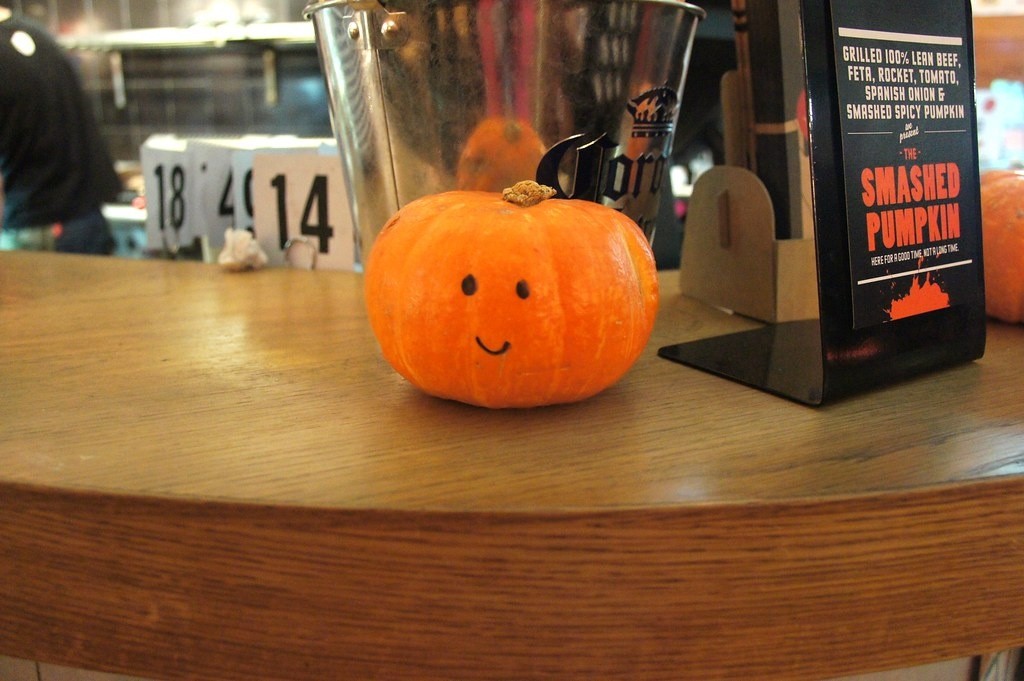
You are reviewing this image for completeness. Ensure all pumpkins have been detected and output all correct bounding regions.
[978,167,1024,324]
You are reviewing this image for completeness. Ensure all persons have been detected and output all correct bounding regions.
[1,1,124,256]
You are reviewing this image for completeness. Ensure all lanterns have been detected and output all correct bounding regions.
[360,181,661,414]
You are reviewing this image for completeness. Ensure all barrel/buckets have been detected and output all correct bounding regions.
[301,0,706,276]
